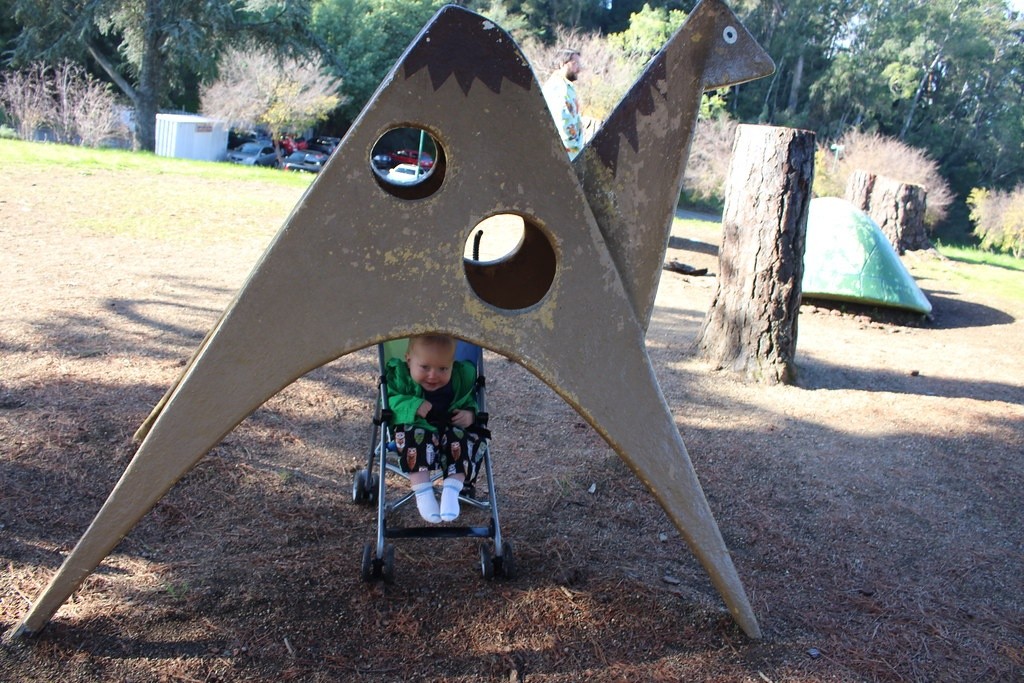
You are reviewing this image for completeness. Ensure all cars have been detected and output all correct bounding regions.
[224,142,284,167]
[375,154,397,170]
[279,149,330,175]
[388,163,427,185]
[231,128,342,155]
[391,148,435,170]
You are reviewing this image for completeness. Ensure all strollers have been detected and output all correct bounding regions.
[351,335,516,583]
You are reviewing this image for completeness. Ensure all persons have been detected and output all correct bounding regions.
[382,332,478,524]
